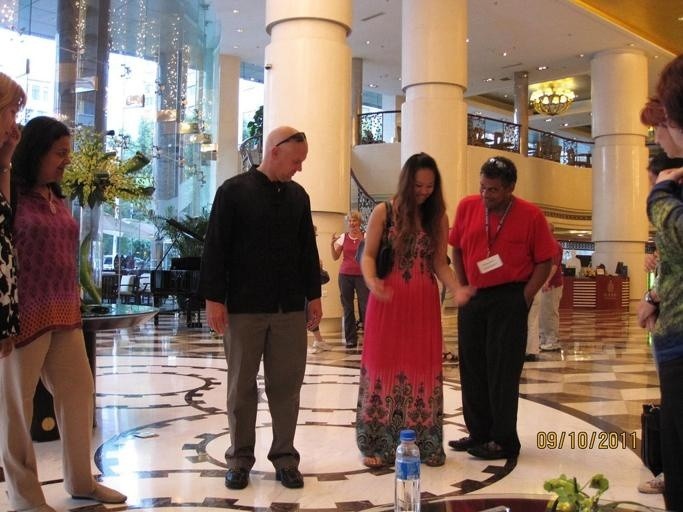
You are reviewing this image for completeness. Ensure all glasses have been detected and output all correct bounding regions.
[488,157,513,180]
[274,133,306,147]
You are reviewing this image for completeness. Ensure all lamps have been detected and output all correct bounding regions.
[528,85,574,116]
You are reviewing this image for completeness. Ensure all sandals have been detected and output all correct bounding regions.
[443,352,458,362]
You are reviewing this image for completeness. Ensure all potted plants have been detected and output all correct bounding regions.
[26,122,159,441]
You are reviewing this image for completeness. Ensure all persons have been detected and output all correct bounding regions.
[354,150,481,470]
[632,49,683,512]
[0,113,128,511]
[638,155,683,494]
[328,209,368,348]
[195,122,323,491]
[306,223,335,355]
[638,97,683,273]
[537,219,565,353]
[0,72,27,361]
[447,154,562,463]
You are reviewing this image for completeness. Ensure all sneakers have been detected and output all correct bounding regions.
[347,343,357,348]
[639,472,664,493]
[73,482,127,503]
[539,343,561,350]
[313,340,332,351]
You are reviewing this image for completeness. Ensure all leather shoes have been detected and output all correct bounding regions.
[226,468,250,489]
[524,354,535,361]
[449,437,520,458]
[276,467,304,488]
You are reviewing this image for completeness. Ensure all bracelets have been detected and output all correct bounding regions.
[0,159,12,179]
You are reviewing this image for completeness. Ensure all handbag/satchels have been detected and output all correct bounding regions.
[641,403,663,469]
[354,239,392,278]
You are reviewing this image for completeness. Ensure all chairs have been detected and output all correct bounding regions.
[463,114,592,169]
[101,257,206,329]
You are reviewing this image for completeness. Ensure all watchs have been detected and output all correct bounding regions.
[642,288,661,310]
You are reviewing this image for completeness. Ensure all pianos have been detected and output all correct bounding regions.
[150,219,205,328]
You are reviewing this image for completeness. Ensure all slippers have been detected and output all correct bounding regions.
[427,460,444,467]
[364,456,385,468]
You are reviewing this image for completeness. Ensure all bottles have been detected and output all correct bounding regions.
[392,427,421,511]
